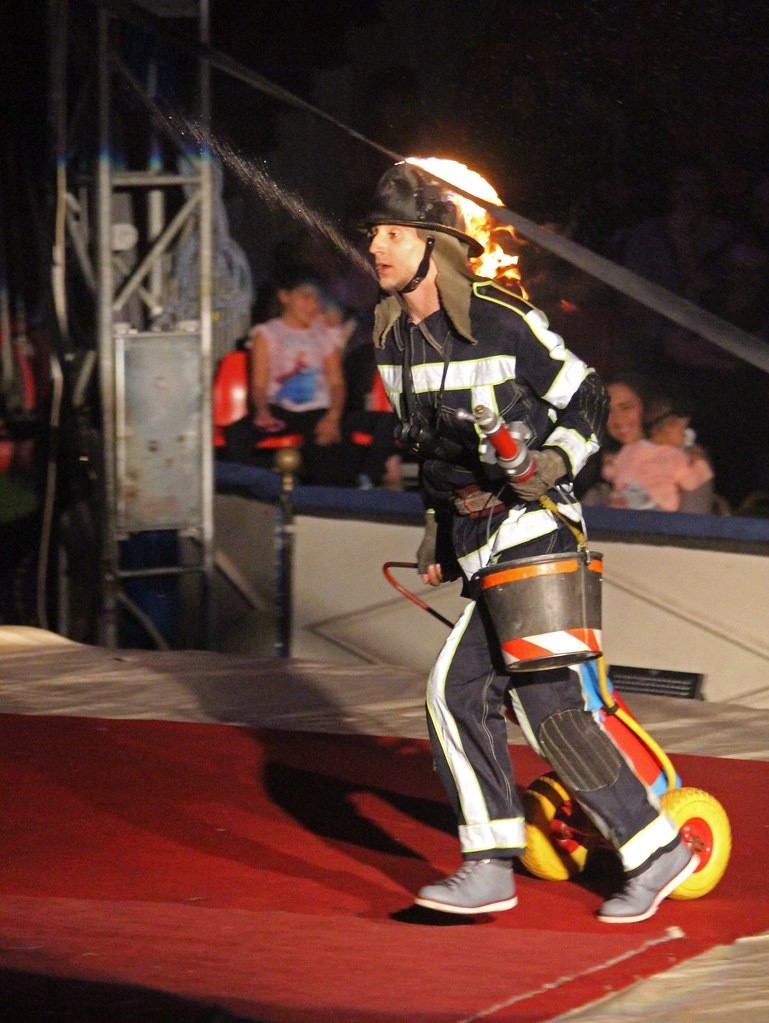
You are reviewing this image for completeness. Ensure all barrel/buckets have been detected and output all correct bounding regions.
[472,484,604,672]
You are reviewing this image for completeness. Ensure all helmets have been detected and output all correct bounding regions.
[352,163,484,260]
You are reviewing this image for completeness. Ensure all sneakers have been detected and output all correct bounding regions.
[414,859,520,916]
[595,841,701,924]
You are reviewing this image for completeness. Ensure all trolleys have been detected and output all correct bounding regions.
[382,560,734,901]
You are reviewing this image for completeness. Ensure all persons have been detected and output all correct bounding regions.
[364,161,704,927]
[212,272,728,519]
